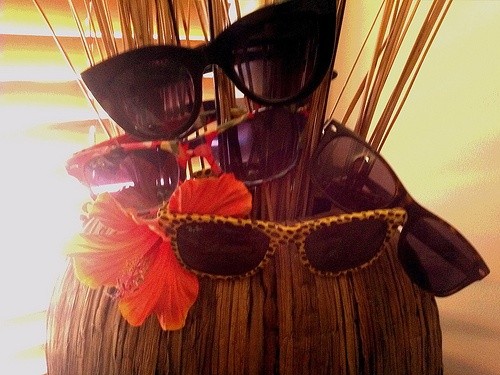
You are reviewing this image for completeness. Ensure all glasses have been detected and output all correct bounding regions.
[308,118,491,298]
[64,98,310,212]
[79,0,339,141]
[157,201,408,283]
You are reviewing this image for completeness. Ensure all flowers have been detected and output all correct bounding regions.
[72,171,252,332]
